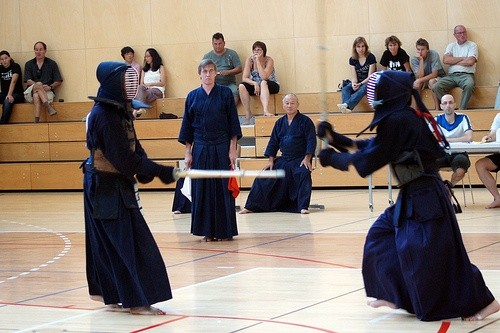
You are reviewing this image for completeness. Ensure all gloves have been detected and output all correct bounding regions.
[156,165,176,184]
[317,121,353,153]
[319,147,352,171]
[136,156,155,184]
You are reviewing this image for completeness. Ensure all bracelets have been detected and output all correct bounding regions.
[359,81,362,85]
[50,86,53,90]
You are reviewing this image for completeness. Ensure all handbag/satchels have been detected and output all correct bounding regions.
[337,80,351,92]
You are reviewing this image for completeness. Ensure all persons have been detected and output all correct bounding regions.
[429,94,473,187]
[409,38,446,110]
[337,37,377,113]
[239,93,317,214]
[0,50,25,124]
[121,47,140,81]
[80,62,180,315]
[238,41,280,125]
[432,25,478,110]
[23,42,63,123]
[379,36,412,74]
[178,59,242,241]
[317,70,500,322]
[201,33,242,105]
[475,112,500,208]
[133,48,167,118]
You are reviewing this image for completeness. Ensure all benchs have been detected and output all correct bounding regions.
[0,83,500,192]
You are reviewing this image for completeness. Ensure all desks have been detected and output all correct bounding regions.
[366,141,500,212]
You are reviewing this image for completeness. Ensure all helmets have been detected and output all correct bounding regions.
[367,70,413,131]
[96,61,138,102]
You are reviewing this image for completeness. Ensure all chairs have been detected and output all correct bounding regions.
[439,167,474,207]
